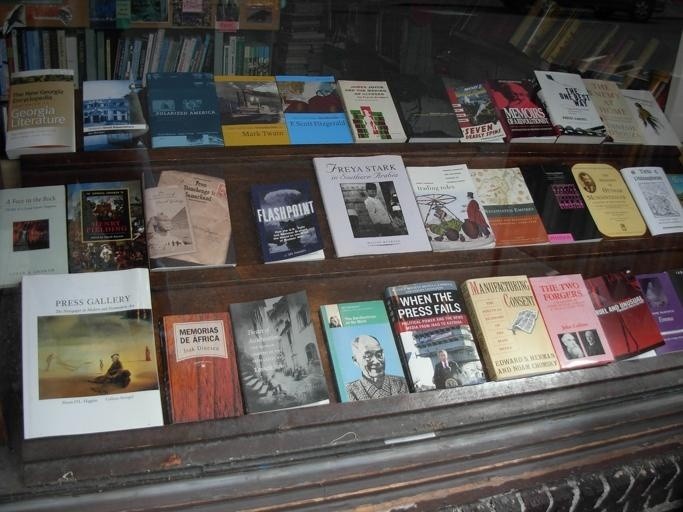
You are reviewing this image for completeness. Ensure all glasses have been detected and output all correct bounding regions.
[355,349,384,360]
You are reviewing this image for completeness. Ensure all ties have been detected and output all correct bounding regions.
[444,362,447,367]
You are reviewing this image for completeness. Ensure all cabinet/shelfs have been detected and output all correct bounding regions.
[0,0,683,512]
